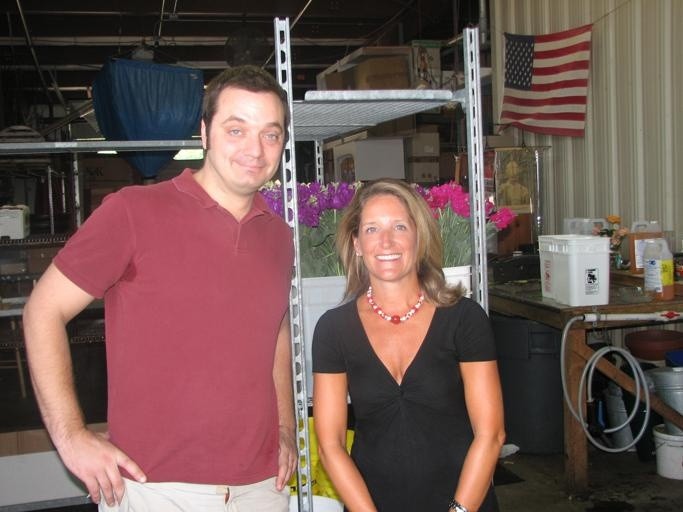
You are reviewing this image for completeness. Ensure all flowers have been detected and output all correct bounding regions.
[261,175,518,276]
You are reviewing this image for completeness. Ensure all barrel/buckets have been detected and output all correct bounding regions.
[615,362,670,462]
[622,217,665,276]
[598,385,639,454]
[646,361,683,435]
[649,420,682,482]
[623,328,682,361]
[638,234,678,304]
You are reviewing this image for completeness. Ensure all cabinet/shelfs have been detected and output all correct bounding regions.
[1,16,308,511]
[276,11,500,512]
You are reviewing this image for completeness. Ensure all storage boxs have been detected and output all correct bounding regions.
[315,44,441,89]
[322,116,440,178]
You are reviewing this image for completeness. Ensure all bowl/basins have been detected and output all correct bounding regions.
[625,330,683,361]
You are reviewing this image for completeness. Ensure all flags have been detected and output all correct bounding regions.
[498,24,590,139]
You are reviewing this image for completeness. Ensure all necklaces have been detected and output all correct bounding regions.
[365,286,425,323]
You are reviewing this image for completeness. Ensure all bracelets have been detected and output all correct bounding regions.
[448,495,470,512]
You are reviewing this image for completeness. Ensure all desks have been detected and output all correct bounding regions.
[489,271,680,496]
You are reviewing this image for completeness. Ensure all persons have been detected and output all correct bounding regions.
[312,179,506,512]
[417,45,428,81]
[22,65,299,510]
[498,158,530,206]
